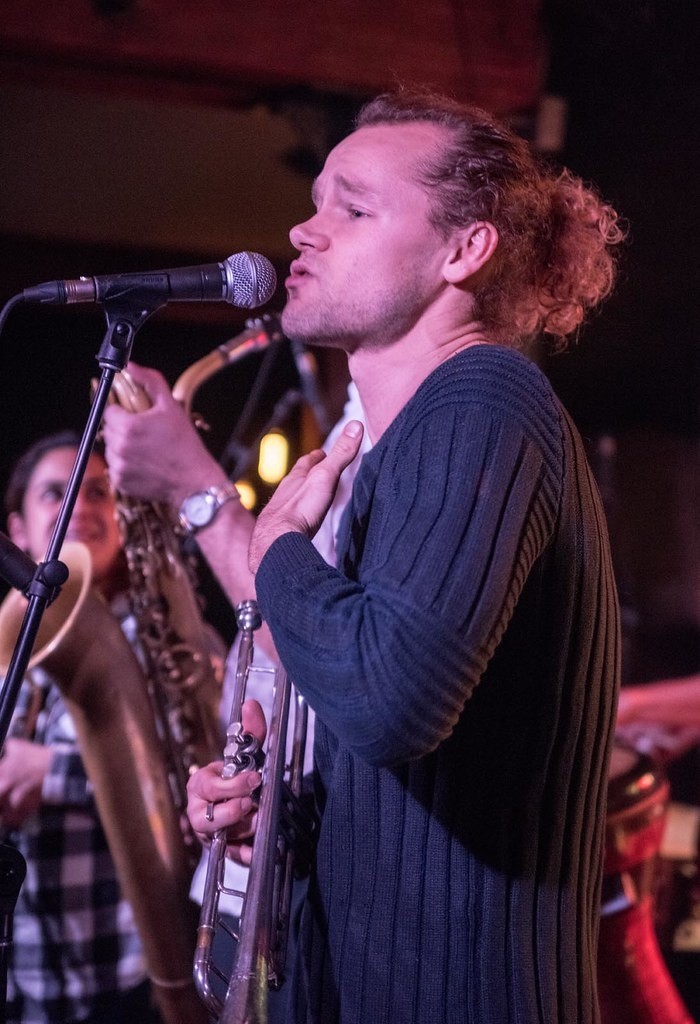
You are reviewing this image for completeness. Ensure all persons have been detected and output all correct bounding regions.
[237,82,632,1024]
[87,350,381,1024]
[0,433,227,1023]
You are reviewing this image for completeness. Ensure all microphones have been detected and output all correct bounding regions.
[22,251,277,309]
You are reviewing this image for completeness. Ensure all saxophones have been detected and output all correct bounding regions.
[0,307,285,1024]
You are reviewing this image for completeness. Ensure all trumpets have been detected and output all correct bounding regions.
[188,599,314,1023]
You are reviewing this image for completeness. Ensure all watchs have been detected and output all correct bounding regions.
[168,477,243,544]
